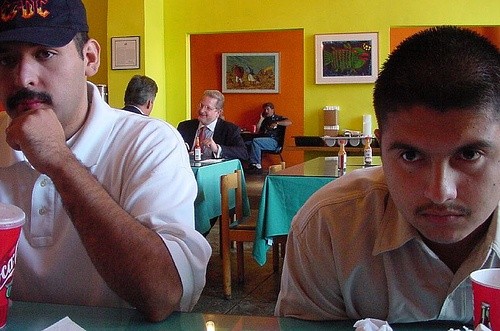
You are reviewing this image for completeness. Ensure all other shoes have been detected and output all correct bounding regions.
[248,164,262,174]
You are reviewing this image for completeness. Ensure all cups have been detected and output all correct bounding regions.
[0,203,25,328]
[469,268,500,331]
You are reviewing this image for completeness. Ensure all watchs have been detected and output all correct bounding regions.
[275,121,277,124]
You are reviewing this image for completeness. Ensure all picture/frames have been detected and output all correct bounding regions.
[314,32,378,85]
[221,52,281,94]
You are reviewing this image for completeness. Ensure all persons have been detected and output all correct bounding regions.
[177,90,248,237]
[0,0,212,322]
[250,102,292,175]
[121,75,158,116]
[275,25,500,322]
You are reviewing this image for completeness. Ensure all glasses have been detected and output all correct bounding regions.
[198,103,214,111]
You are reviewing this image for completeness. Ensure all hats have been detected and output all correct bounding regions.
[0,0,89,47]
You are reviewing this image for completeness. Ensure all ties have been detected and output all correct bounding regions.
[199,127,208,154]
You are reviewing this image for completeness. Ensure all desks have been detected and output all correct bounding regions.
[191,158,251,238]
[253,156,382,272]
[291,134,382,163]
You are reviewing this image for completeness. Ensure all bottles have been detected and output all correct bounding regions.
[364,139,372,163]
[478,302,492,329]
[338,140,347,172]
[194,137,202,161]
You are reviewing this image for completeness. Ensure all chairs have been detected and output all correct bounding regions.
[219,169,284,300]
[259,127,287,172]
